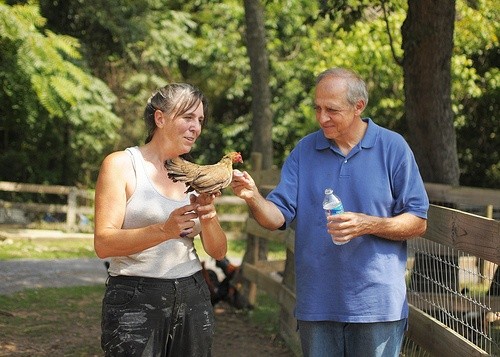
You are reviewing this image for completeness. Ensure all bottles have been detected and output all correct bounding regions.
[323,188,351,245]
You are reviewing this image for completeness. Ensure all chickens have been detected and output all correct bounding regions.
[164,151,245,196]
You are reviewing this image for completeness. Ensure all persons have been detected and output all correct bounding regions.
[227,65,430,357]
[94,84,227,357]
